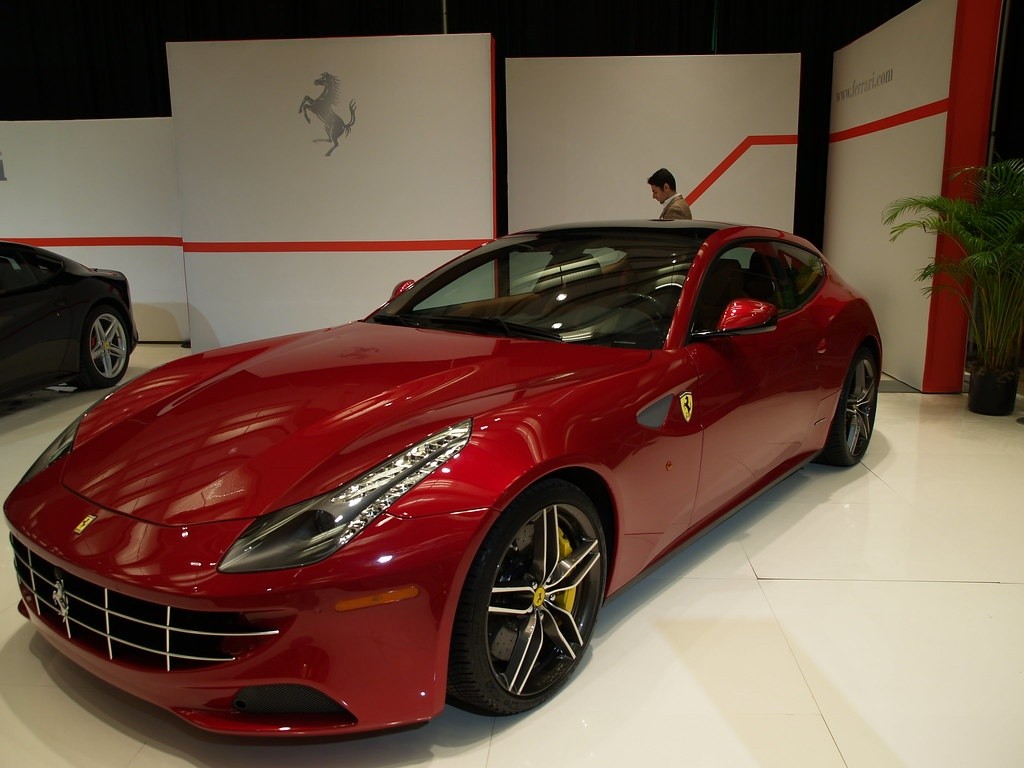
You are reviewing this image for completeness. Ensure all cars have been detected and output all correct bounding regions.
[0,241,139,396]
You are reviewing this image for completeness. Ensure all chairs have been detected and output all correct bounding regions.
[749,251,797,308]
[505,253,602,324]
[665,259,743,329]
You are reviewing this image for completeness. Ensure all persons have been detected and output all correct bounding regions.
[647,168,693,220]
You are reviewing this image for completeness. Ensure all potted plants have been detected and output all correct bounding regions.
[883,148,1024,416]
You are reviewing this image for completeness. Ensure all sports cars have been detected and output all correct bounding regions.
[4,219,883,737]
